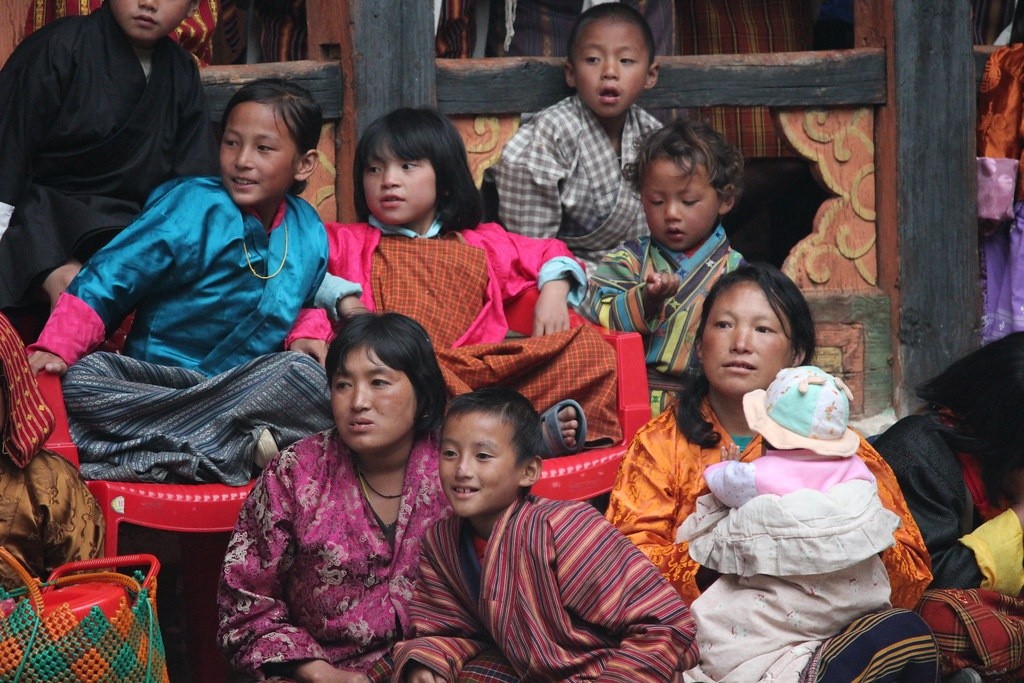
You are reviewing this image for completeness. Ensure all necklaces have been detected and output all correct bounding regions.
[244,217,288,279]
[360,472,401,499]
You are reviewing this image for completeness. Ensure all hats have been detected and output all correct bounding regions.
[743,364,860,457]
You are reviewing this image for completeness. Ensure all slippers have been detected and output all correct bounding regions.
[537,398,586,457]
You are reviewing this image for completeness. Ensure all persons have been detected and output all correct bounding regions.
[0,0,1024,683]
[218,312,454,683]
[481,2,664,281]
[368,385,701,682]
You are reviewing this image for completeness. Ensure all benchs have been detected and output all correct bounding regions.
[38,317,653,682]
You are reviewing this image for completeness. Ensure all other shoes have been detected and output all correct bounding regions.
[252,427,279,469]
